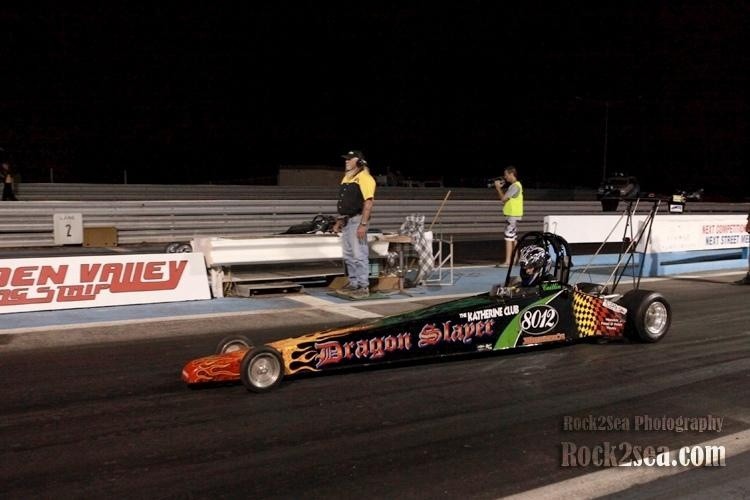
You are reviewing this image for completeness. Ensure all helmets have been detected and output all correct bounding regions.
[518,245,555,287]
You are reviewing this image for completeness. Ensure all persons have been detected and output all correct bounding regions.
[0,172,18,201]
[492,162,525,269]
[330,149,377,300]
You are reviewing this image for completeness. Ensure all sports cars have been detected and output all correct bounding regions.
[174,189,706,390]
[164,213,397,255]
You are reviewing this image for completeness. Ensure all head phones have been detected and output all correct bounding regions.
[352,151,363,167]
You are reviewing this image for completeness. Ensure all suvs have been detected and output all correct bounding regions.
[595,173,641,200]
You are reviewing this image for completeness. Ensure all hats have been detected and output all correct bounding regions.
[341,150,363,159]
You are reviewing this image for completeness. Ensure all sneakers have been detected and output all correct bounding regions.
[336,282,370,299]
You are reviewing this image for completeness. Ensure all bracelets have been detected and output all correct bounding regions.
[360,221,368,227]
